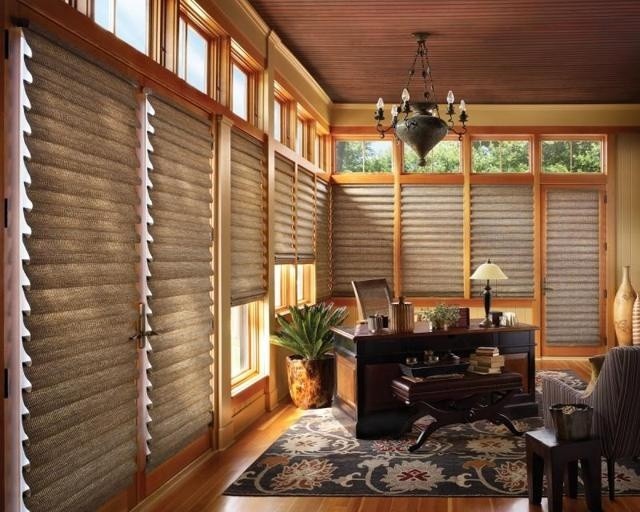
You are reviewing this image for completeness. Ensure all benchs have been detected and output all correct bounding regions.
[390,371,526,453]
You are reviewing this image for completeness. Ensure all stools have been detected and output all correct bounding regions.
[525,426,603,512]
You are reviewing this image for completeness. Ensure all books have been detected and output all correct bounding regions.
[465,346,506,376]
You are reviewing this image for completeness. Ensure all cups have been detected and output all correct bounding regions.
[488,311,503,326]
[368,315,383,334]
[405,349,441,367]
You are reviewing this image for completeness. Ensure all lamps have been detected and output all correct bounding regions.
[468,259,508,328]
[373,32,468,167]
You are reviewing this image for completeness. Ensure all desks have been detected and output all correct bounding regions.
[328,319,539,440]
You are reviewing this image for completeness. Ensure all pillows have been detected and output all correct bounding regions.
[583,355,605,397]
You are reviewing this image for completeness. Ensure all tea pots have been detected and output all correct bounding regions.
[442,353,461,367]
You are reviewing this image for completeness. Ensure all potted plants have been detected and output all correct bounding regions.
[270,299,351,409]
[414,304,460,333]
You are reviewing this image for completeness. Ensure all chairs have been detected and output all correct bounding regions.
[538,346,640,500]
[351,279,394,323]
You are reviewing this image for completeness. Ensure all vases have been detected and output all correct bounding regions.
[613,266,637,346]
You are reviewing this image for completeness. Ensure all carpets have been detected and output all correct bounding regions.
[221,369,640,497]
